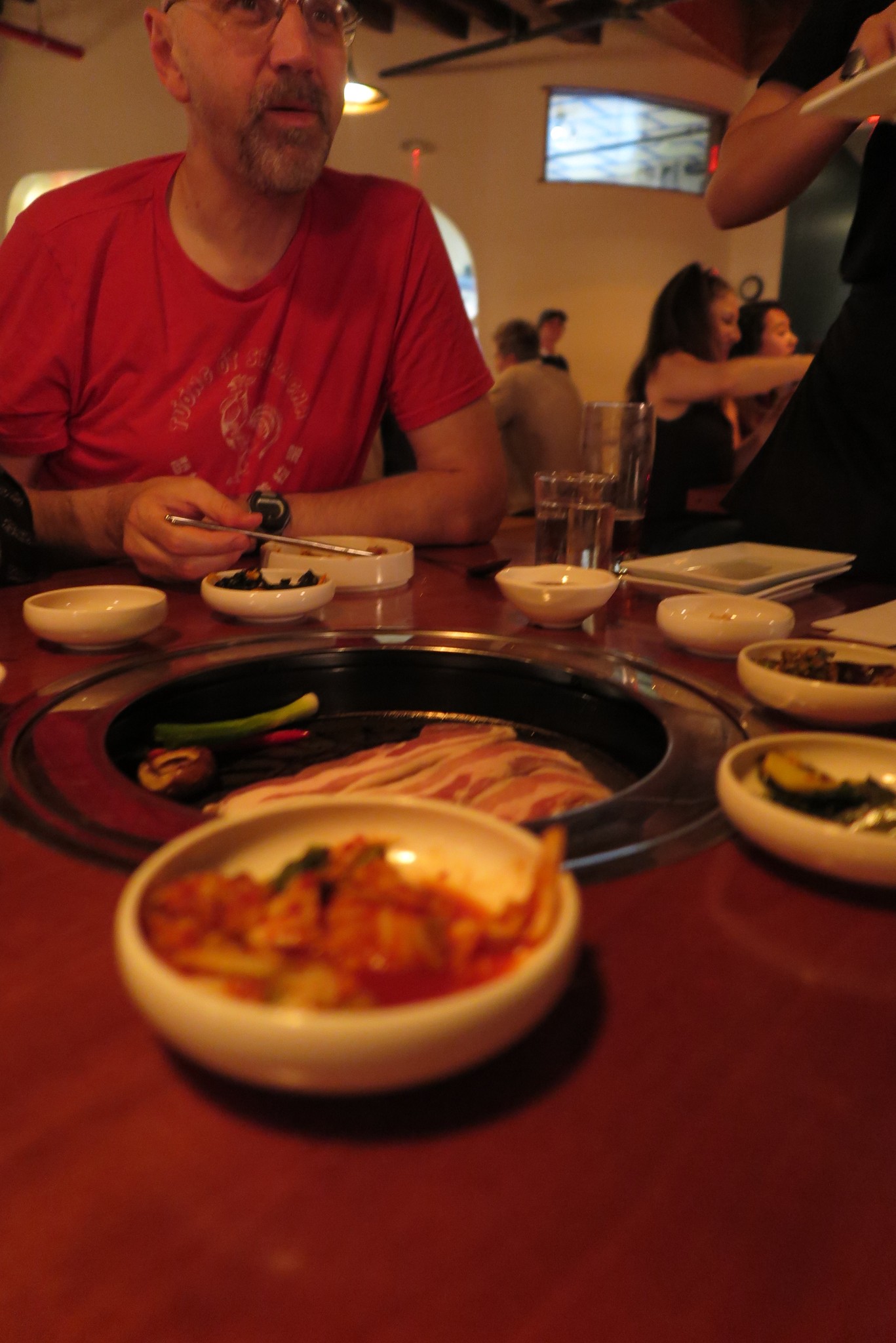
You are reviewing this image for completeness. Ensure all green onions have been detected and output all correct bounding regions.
[153,691,319,746]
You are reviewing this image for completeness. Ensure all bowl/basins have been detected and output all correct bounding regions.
[495,563,619,630]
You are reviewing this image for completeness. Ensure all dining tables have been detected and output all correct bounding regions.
[1,526,896,1343]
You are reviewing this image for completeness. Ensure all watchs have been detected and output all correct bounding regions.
[248,489,291,551]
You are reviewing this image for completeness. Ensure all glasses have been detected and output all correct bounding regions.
[164,0,362,48]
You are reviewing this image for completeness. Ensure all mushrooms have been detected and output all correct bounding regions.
[138,746,212,796]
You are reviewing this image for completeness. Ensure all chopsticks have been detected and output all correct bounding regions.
[164,515,377,558]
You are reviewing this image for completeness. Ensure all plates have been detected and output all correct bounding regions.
[657,591,795,659]
[22,585,167,651]
[799,49,896,123]
[257,532,415,592]
[715,732,896,893]
[108,797,583,1100]
[619,541,856,604]
[738,637,895,728]
[199,568,336,624]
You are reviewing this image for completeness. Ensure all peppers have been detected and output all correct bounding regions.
[239,729,310,744]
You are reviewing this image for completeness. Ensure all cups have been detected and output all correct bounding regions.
[576,402,658,557]
[533,471,616,579]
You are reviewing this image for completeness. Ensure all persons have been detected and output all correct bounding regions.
[0,0,505,581]
[489,319,584,513]
[535,309,569,370]
[620,263,813,552]
[704,0,896,595]
[718,301,799,476]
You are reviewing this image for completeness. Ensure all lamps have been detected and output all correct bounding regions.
[343,48,390,116]
